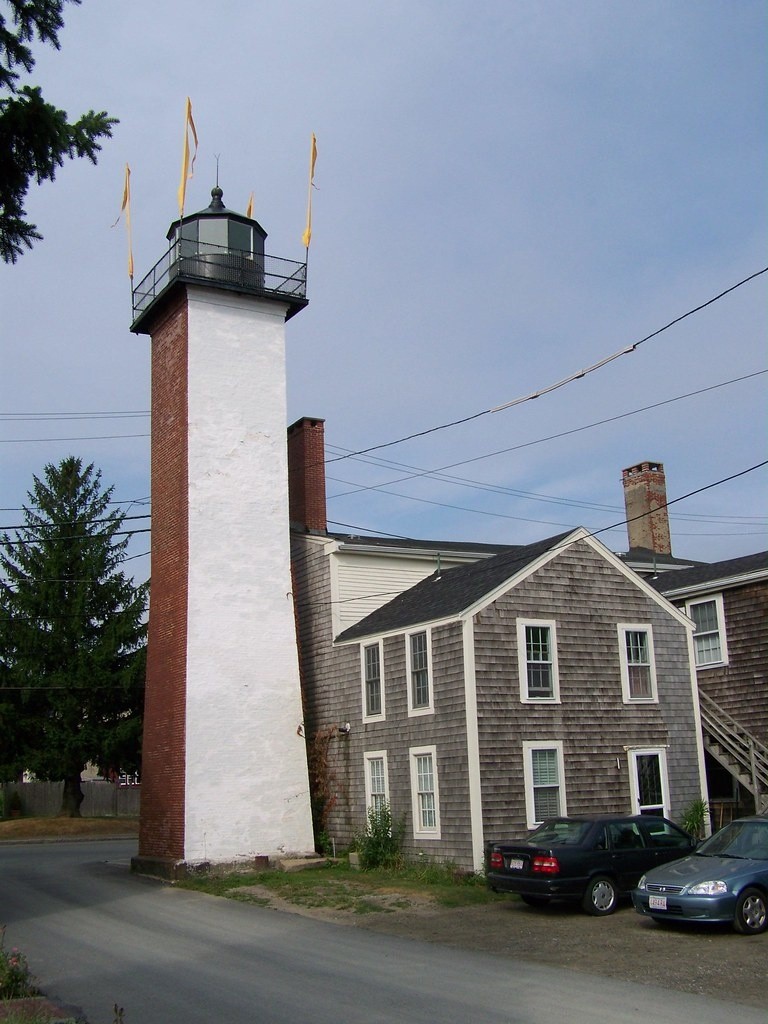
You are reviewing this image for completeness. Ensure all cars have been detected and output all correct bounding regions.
[630,815,768,937]
[485,816,704,916]
